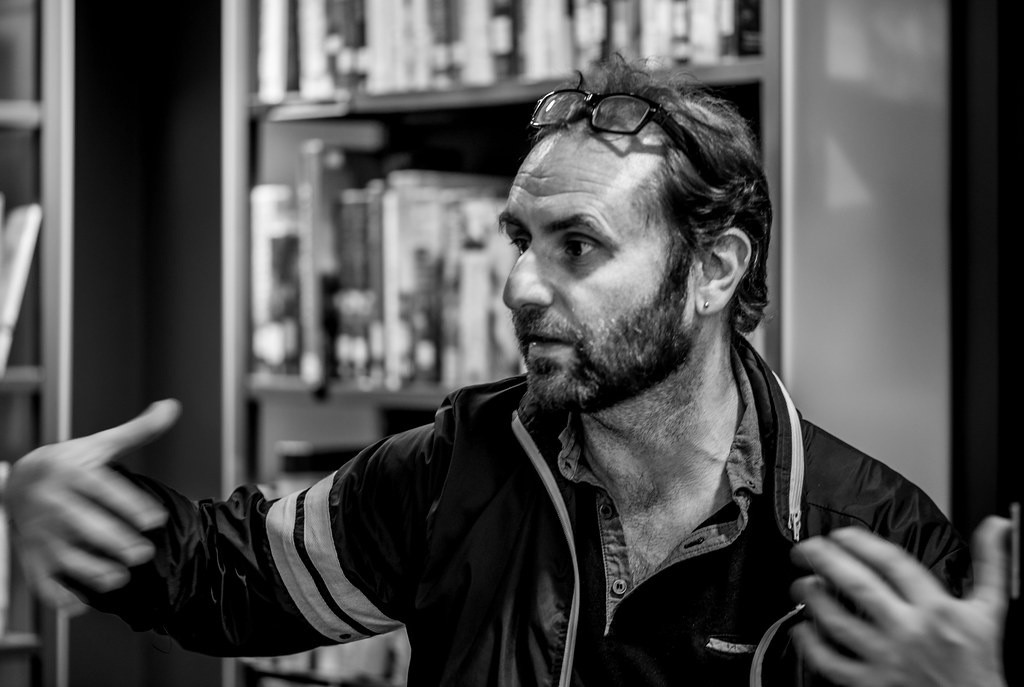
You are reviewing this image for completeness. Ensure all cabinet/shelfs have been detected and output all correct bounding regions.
[220,0,954,687]
[0,0,77,687]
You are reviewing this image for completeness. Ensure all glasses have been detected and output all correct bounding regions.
[530,89,721,190]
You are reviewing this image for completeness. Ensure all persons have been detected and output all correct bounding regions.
[4,77,1010,685]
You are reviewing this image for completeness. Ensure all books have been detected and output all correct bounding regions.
[251,1,769,106]
[251,142,527,392]
